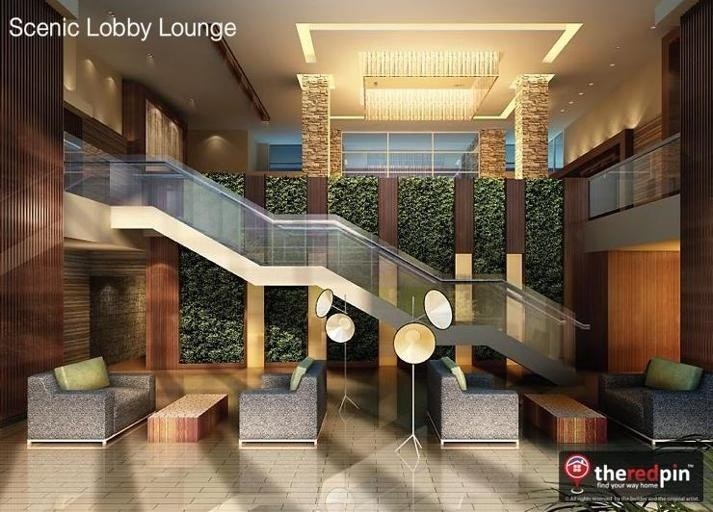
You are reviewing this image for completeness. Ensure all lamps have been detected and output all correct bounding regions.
[313,285,362,414]
[360,49,500,121]
[391,288,453,461]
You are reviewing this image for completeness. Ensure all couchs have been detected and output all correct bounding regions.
[25,356,155,448]
[236,354,328,449]
[597,354,712,451]
[424,355,520,450]
